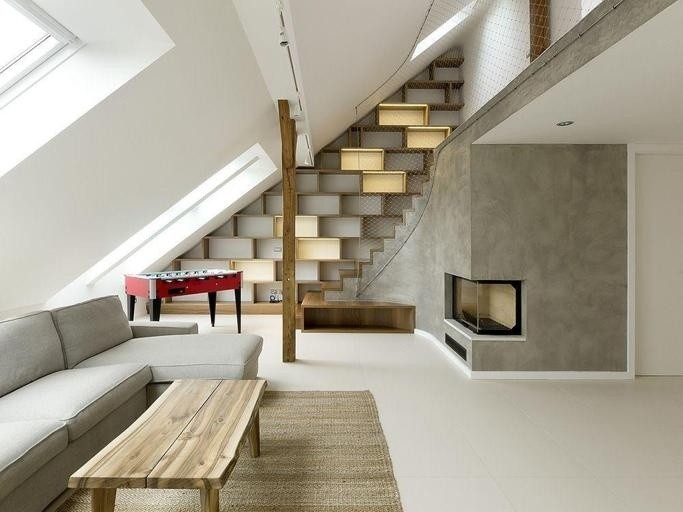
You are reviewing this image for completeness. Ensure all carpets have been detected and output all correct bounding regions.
[41,389,404,512]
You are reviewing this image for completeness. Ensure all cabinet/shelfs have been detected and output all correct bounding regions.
[145,56,464,314]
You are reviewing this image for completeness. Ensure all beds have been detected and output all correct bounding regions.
[50,293,263,410]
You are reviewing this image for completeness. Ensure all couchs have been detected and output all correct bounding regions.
[0,311,153,512]
